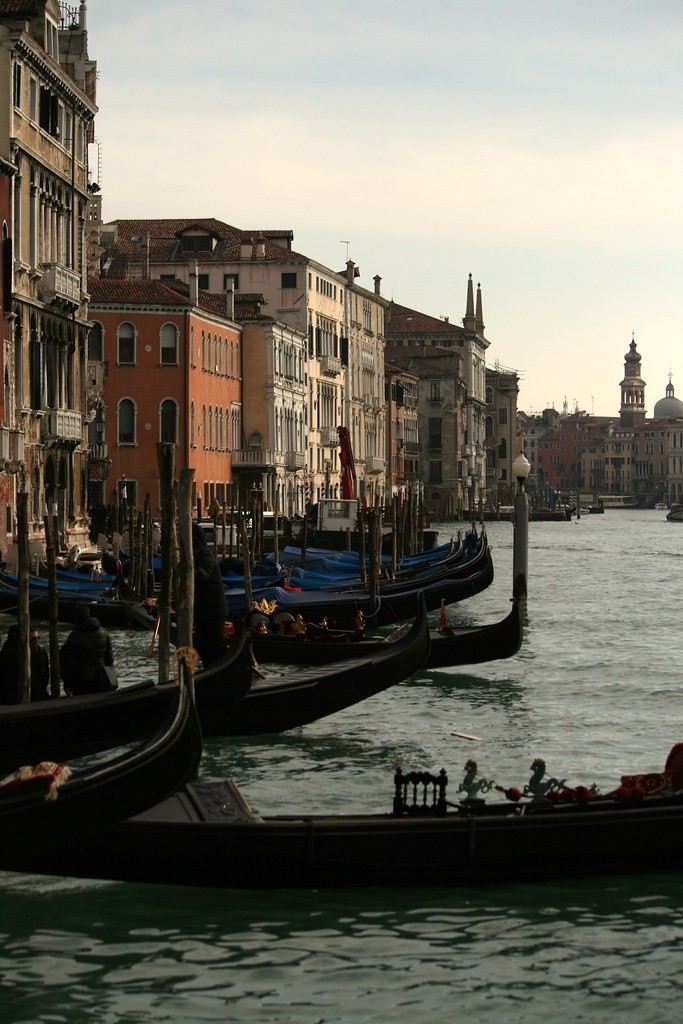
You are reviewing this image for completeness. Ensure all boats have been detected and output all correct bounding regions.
[1,630,256,776]
[655,503,668,510]
[0,650,205,848]
[666,503,683,520]
[206,588,431,740]
[0,517,495,629]
[456,508,567,521]
[118,573,523,669]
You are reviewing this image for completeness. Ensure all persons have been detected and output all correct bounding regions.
[0,624,50,705]
[59,604,119,697]
[101,537,130,589]
